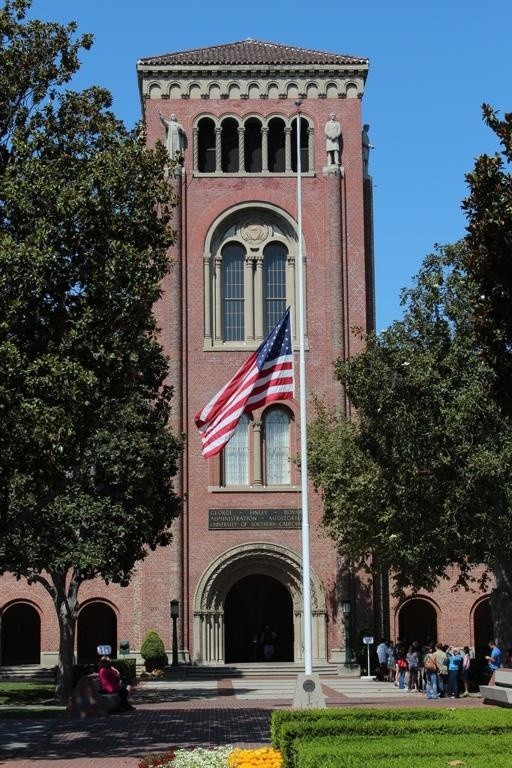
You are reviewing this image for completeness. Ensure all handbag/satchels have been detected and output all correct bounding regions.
[424,655,438,672]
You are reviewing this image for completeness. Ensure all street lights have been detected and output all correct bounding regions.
[168,600,180,666]
[341,595,353,665]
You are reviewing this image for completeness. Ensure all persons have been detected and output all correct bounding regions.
[98,656,137,711]
[325,112,342,165]
[362,124,375,175]
[157,108,188,164]
[110,665,136,708]
[373,632,512,700]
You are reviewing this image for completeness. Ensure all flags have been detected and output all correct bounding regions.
[192,298,297,462]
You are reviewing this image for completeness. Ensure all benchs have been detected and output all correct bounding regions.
[68,673,119,715]
[478,668,511,705]
